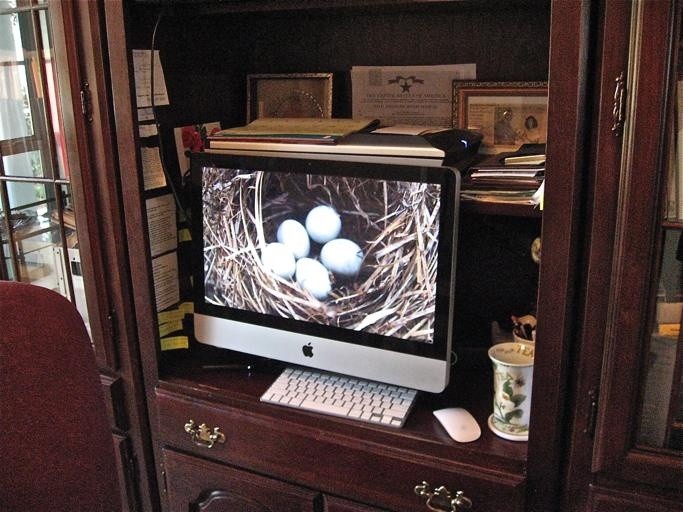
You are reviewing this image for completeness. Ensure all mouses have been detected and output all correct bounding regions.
[432,405,482,444]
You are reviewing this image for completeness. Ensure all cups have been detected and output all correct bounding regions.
[512,329,534,344]
[487,342,535,442]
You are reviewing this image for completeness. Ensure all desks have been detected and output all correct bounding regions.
[0,214,67,297]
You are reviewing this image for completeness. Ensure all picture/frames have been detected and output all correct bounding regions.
[246,72,332,122]
[451,78,550,157]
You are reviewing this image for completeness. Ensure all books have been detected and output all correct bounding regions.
[463,143,547,190]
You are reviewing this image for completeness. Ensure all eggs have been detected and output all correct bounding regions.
[323,240,364,277]
[305,205,341,240]
[261,243,296,279]
[277,219,310,257]
[296,257,331,298]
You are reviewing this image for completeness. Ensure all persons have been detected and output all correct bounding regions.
[517,115,539,141]
[494,107,519,145]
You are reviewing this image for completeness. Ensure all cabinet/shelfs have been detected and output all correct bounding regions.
[73,0,591,511]
[557,2,683,512]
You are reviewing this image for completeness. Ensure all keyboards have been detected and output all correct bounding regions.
[259,367,417,431]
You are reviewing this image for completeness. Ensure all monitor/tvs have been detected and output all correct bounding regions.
[194,153,463,395]
